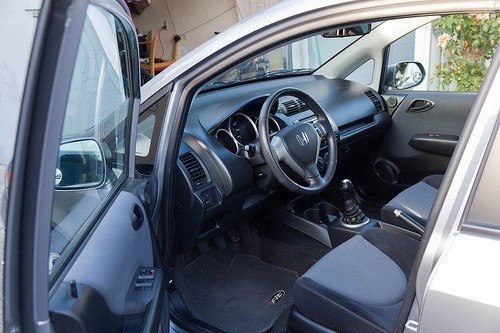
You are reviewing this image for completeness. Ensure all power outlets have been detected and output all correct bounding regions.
[163,20,168,30]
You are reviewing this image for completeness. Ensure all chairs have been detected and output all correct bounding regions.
[285,226,421,333]
[381,175,445,233]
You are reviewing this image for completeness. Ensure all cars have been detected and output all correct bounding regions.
[0,1,500,333]
